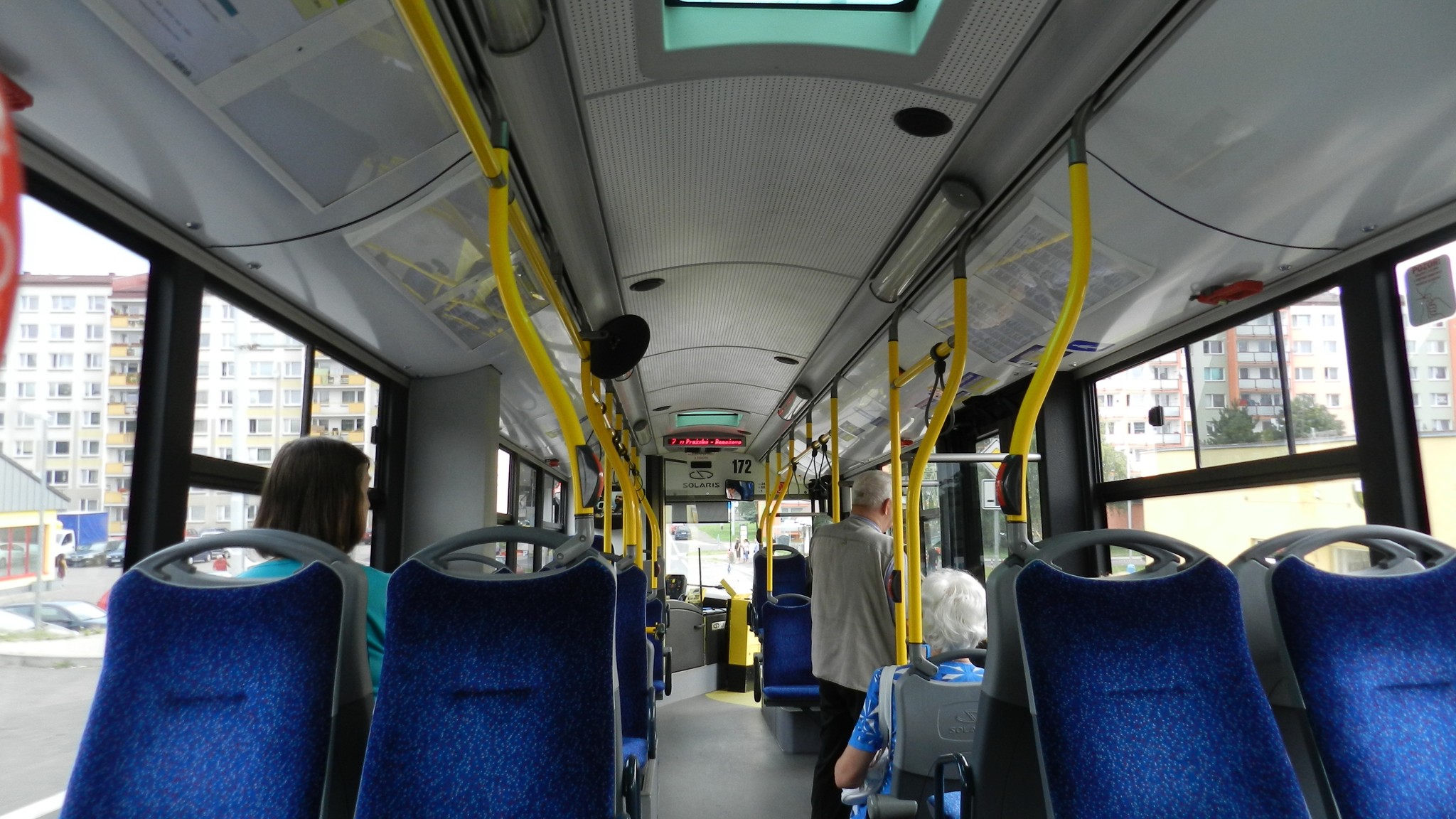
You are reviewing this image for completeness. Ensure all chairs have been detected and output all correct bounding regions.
[749,522,1456,819]
[56,523,671,819]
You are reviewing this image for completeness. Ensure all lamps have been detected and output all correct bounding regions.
[775,384,812,423]
[475,0,549,62]
[634,421,652,446]
[866,178,980,304]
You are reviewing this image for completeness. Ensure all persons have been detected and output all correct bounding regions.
[234,437,394,703]
[734,537,758,563]
[835,568,987,819]
[726,484,741,500]
[212,555,232,571]
[808,470,908,819]
[55,554,68,579]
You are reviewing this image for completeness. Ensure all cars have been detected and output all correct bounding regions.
[364,528,372,545]
[0,527,128,568]
[181,527,230,566]
[674,525,691,540]
[669,523,684,535]
[0,587,111,638]
